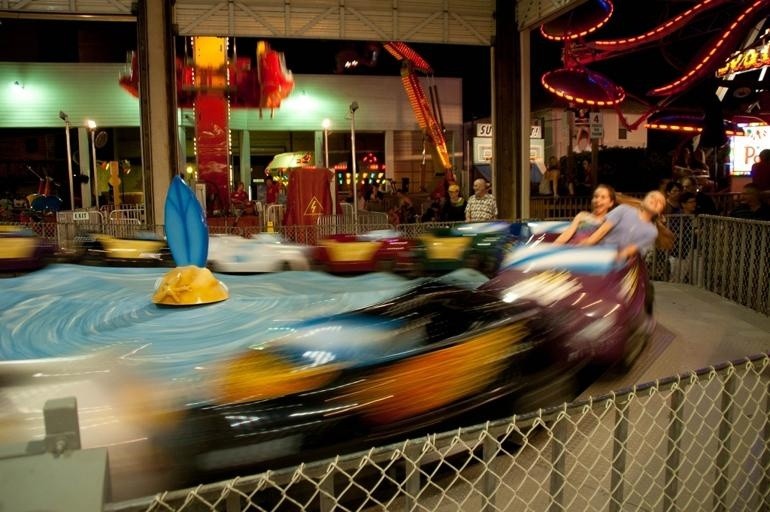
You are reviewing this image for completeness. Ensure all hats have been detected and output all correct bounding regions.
[448,184,459,192]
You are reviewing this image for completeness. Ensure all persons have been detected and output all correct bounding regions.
[539,149,770,287]
[363,41,386,75]
[575,109,589,144]
[346,178,498,222]
[230,176,279,210]
[21,209,55,237]
